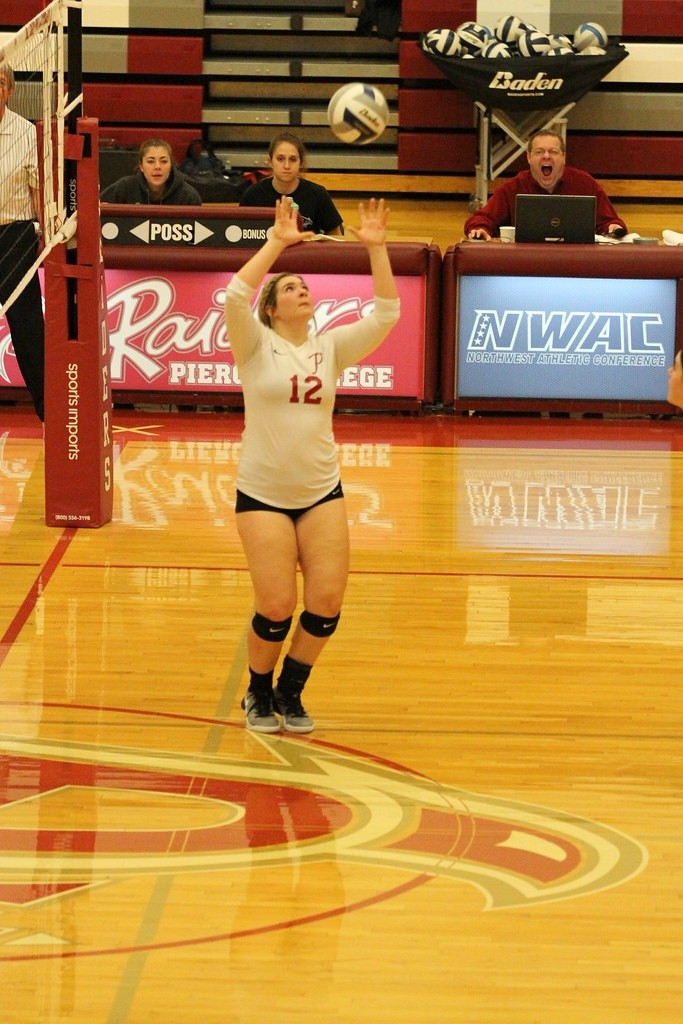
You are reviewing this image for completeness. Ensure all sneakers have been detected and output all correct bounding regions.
[273,680,314,734]
[244,679,281,734]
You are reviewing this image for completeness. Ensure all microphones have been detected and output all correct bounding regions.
[601,228,625,238]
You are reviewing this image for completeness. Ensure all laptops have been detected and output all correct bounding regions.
[515,194,597,244]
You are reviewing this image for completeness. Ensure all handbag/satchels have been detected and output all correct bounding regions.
[179,138,224,179]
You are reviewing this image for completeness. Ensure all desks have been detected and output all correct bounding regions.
[0,241,683,415]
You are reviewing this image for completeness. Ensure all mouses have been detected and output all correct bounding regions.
[473,234,483,240]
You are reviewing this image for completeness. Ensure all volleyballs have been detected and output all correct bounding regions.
[422,27,462,58]
[462,23,607,59]
[480,42,513,59]
[516,29,550,58]
[494,14,528,48]
[327,82,390,145]
[572,21,608,51]
[455,20,490,56]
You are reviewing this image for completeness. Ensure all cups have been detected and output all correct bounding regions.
[500,227,515,244]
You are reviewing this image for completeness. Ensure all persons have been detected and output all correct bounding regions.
[100,139,202,205]
[356,0,403,42]
[224,195,401,733]
[463,129,628,242]
[0,60,46,421]
[666,349,683,409]
[240,134,344,235]
